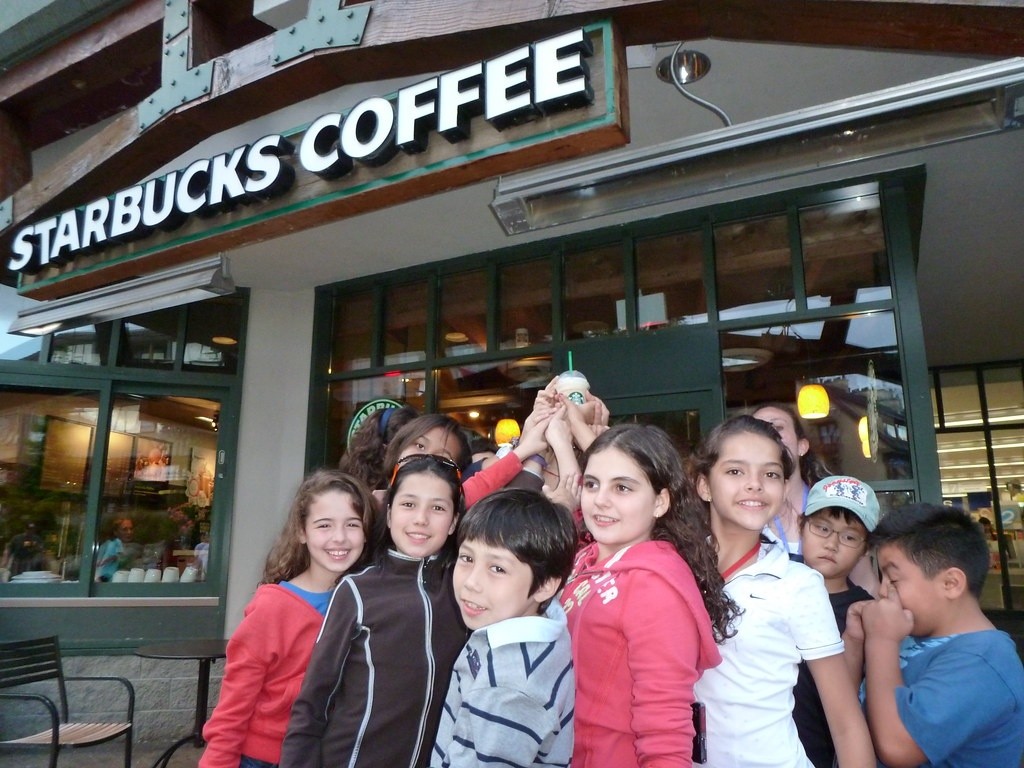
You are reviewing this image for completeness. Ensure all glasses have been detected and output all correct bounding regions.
[807,519,870,549]
[390,454,462,507]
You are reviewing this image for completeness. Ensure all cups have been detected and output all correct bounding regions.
[552,370,590,407]
[113,567,198,584]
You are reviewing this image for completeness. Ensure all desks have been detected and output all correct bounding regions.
[135,639,229,768]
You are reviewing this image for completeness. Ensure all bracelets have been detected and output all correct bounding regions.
[529,454,547,467]
[544,469,558,478]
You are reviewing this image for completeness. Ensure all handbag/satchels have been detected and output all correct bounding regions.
[691,702,707,764]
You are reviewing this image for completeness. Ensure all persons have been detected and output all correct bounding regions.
[751,404,881,600]
[199,375,722,768]
[0,522,39,582]
[430,471,583,768]
[685,414,878,768]
[95,518,133,582]
[841,501,1024,768]
[792,475,880,768]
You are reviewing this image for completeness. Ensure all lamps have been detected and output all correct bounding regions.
[495,361,521,446]
[798,337,829,420]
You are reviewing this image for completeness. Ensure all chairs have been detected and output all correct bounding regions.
[0,635,135,768]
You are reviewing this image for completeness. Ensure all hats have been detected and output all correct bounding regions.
[805,475,881,532]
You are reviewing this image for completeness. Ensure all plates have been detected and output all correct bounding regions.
[12,571,61,583]
